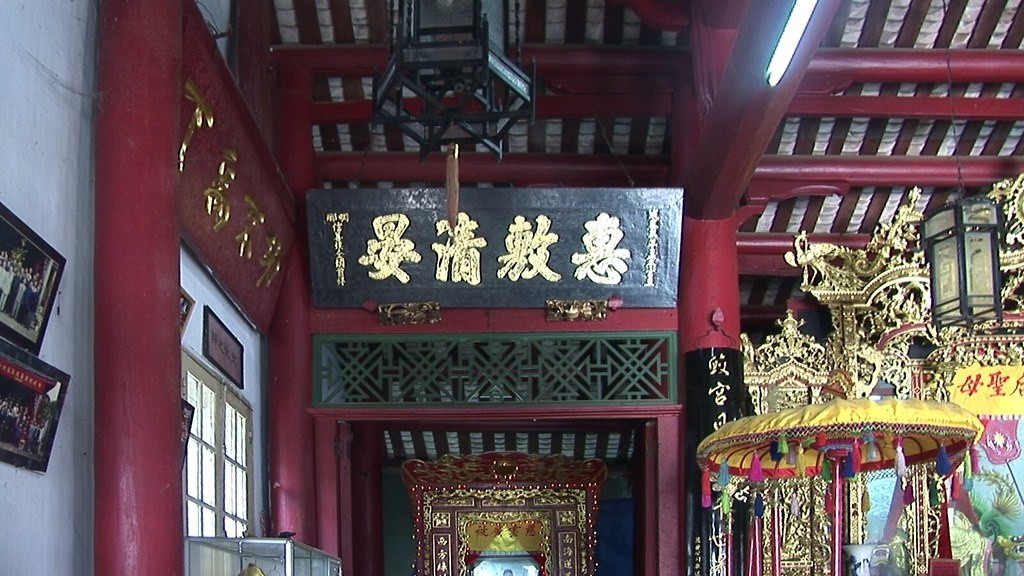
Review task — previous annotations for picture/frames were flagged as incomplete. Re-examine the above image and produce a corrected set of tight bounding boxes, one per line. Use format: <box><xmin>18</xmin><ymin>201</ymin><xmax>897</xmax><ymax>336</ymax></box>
<box><xmin>0</xmin><ymin>201</ymin><xmax>67</xmax><ymax>357</ymax></box>
<box><xmin>181</xmin><ymin>395</ymin><xmax>195</xmax><ymax>467</ymax></box>
<box><xmin>179</xmin><ymin>286</ymin><xmax>196</xmax><ymax>337</ymax></box>
<box><xmin>0</xmin><ymin>339</ymin><xmax>71</xmax><ymax>472</ymax></box>
<box><xmin>201</xmin><ymin>305</ymin><xmax>244</xmax><ymax>387</ymax></box>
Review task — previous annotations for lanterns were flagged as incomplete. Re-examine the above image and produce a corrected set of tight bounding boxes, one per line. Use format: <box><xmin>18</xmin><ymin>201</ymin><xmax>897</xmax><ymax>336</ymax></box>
<box><xmin>922</xmin><ymin>200</ymin><xmax>1004</xmax><ymax>334</ymax></box>
<box><xmin>372</xmin><ymin>0</ymin><xmax>536</xmax><ymax>232</ymax></box>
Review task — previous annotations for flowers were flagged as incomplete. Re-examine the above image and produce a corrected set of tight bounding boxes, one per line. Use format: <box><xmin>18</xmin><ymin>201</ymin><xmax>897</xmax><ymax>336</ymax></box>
<box><xmin>843</xmin><ymin>543</ymin><xmax>877</xmax><ymax>576</ymax></box>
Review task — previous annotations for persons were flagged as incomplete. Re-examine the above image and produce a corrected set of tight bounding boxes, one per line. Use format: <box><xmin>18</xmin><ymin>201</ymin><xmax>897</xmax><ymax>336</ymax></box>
<box><xmin>0</xmin><ymin>249</ymin><xmax>40</xmax><ymax>331</ymax></box>
<box><xmin>0</xmin><ymin>394</ymin><xmax>45</xmax><ymax>454</ymax></box>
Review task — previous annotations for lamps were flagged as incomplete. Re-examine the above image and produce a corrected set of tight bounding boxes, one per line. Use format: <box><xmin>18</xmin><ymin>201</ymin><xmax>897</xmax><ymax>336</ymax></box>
<box><xmin>764</xmin><ymin>0</ymin><xmax>818</xmax><ymax>86</ymax></box>
<box><xmin>918</xmin><ymin>0</ymin><xmax>1005</xmax><ymax>330</ymax></box>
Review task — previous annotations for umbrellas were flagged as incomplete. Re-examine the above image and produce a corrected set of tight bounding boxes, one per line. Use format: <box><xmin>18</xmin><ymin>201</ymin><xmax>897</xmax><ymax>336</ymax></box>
<box><xmin>697</xmin><ymin>395</ymin><xmax>985</xmax><ymax>575</ymax></box>
